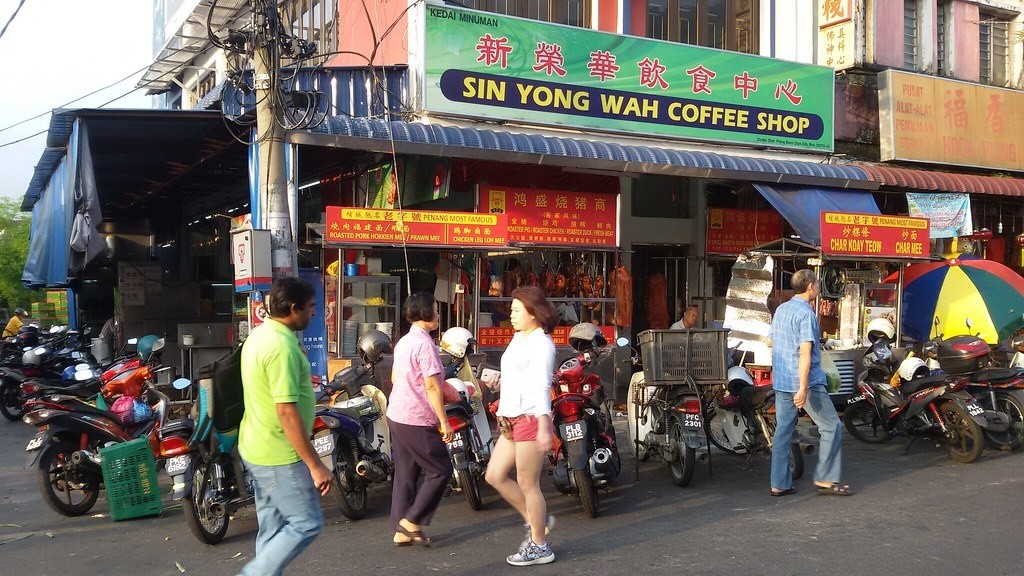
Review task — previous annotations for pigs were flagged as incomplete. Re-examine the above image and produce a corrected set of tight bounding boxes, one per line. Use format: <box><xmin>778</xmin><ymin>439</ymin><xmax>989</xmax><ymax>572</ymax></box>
<box><xmin>607</xmin><ymin>267</ymin><xmax>670</xmax><ymax>330</ymax></box>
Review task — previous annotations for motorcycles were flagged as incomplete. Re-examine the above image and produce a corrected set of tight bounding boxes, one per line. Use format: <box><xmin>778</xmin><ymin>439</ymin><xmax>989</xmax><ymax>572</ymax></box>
<box><xmin>435</xmin><ymin>338</ymin><xmax>491</xmax><ymax>508</ymax></box>
<box><xmin>616</xmin><ymin>337</ymin><xmax>706</xmax><ymax>488</ymax></box>
<box><xmin>923</xmin><ymin>313</ymin><xmax>1024</xmax><ymax>454</ymax></box>
<box><xmin>703</xmin><ymin>347</ymin><xmax>817</xmax><ymax>479</ymax></box>
<box><xmin>0</xmin><ymin>321</ymin><xmax>168</xmax><ymax>518</ymax></box>
<box><xmin>160</xmin><ymin>364</ymin><xmax>255</xmax><ymax>544</ymax></box>
<box><xmin>549</xmin><ymin>338</ymin><xmax>623</xmax><ymax>516</ymax></box>
<box><xmin>308</xmin><ymin>357</ymin><xmax>397</xmax><ymax>518</ymax></box>
<box><xmin>843</xmin><ymin>318</ymin><xmax>989</xmax><ymax>463</ymax></box>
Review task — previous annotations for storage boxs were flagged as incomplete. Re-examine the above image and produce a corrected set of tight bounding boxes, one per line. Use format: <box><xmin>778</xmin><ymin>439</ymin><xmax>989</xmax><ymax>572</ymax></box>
<box><xmin>636</xmin><ymin>328</ymin><xmax>732</xmax><ymax>386</ymax></box>
<box><xmin>935</xmin><ymin>333</ymin><xmax>990</xmax><ymax>374</ymax></box>
<box><xmin>97</xmin><ymin>433</ymin><xmax>163</xmax><ymax>523</ymax></box>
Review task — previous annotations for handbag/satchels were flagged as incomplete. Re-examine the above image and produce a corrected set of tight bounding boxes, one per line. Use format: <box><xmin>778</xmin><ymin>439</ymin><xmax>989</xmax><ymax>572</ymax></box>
<box><xmin>495</xmin><ymin>416</ymin><xmax>521</xmax><ymax>439</ymax></box>
<box><xmin>186</xmin><ymin>337</ymin><xmax>248</xmax><ymax>454</ymax></box>
<box><xmin>96</xmin><ymin>392</ymin><xmax>108</xmax><ymax>411</ymax></box>
<box><xmin>110</xmin><ymin>396</ymin><xmax>153</xmax><ymax>427</ymax></box>
<box><xmin>488</xmin><ymin>273</ymin><xmax>505</xmax><ymax>297</ymax></box>
<box><xmin>890</xmin><ymin>351</ymin><xmax>916</xmax><ymax>388</ymax></box>
<box><xmin>453</xmin><ymin>270</ymin><xmax>472</xmax><ymax>314</ymax></box>
<box><xmin>818</xmin><ymin>345</ymin><xmax>841</xmax><ymax>394</ymax></box>
<box><xmin>433</xmin><ymin>279</ymin><xmax>455</xmax><ymax>305</ymax></box>
<box><xmin>458</xmin><ymin>356</ymin><xmax>482</xmax><ymax>399</ymax></box>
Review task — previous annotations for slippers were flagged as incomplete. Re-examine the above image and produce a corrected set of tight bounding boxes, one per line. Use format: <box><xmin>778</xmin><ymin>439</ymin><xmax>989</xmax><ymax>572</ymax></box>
<box><xmin>770</xmin><ymin>487</ymin><xmax>798</xmax><ymax>496</ymax></box>
<box><xmin>816</xmin><ymin>482</ymin><xmax>855</xmax><ymax>496</ymax></box>
<box><xmin>393</xmin><ymin>524</ymin><xmax>431</xmax><ymax>547</ymax></box>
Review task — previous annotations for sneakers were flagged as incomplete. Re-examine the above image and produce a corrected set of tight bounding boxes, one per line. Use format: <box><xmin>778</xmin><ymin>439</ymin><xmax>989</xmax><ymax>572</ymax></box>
<box><xmin>505</xmin><ymin>540</ymin><xmax>555</xmax><ymax>566</ymax></box>
<box><xmin>518</xmin><ymin>515</ymin><xmax>554</xmax><ymax>552</ymax></box>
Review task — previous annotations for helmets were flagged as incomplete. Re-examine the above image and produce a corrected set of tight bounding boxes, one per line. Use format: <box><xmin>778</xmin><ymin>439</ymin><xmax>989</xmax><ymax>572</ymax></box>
<box><xmin>22</xmin><ymin>350</ymin><xmax>40</xmax><ymax>366</ymax></box>
<box><xmin>441</xmin><ymin>327</ymin><xmax>478</xmax><ymax>358</ymax></box>
<box><xmin>65</xmin><ymin>327</ymin><xmax>81</xmax><ymax>340</ymax></box>
<box><xmin>356</xmin><ymin>329</ymin><xmax>394</xmax><ymax>362</ymax></box>
<box><xmin>136</xmin><ymin>335</ymin><xmax>159</xmax><ymax>362</ymax></box>
<box><xmin>866</xmin><ymin>318</ymin><xmax>895</xmax><ymax>344</ymax></box>
<box><xmin>899</xmin><ymin>357</ymin><xmax>930</xmax><ymax>382</ymax></box>
<box><xmin>724</xmin><ymin>366</ymin><xmax>754</xmax><ymax>396</ymax></box>
<box><xmin>568</xmin><ymin>323</ymin><xmax>607</xmax><ymax>351</ymax></box>
<box><xmin>61</xmin><ymin>365</ymin><xmax>76</xmax><ymax>383</ymax></box>
<box><xmin>14</xmin><ymin>308</ymin><xmax>23</xmax><ymax>315</ymax></box>
<box><xmin>444</xmin><ymin>378</ymin><xmax>469</xmax><ymax>403</ymax></box>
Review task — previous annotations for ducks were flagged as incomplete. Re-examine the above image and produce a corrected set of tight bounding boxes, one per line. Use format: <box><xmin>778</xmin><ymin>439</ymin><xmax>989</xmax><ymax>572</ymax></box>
<box><xmin>488</xmin><ymin>251</ymin><xmax>604</xmax><ymax>311</ymax></box>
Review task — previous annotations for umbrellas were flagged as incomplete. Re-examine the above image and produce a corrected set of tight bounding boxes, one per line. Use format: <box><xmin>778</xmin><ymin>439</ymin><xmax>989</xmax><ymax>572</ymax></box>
<box><xmin>868</xmin><ymin>253</ymin><xmax>1024</xmax><ymax>349</ymax></box>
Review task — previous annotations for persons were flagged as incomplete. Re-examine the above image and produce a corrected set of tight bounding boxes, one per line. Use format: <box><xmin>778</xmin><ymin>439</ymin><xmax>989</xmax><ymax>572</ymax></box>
<box><xmin>490</xmin><ymin>258</ymin><xmax>522</xmax><ymax>327</ymax></box>
<box><xmin>766</xmin><ymin>269</ymin><xmax>854</xmax><ymax>495</ymax></box>
<box><xmin>669</xmin><ymin>306</ymin><xmax>698</xmax><ymax>330</ymax></box>
<box><xmin>386</xmin><ymin>291</ymin><xmax>454</xmax><ymax>546</ymax></box>
<box><xmin>233</xmin><ymin>276</ymin><xmax>335</xmax><ymax>576</ymax></box>
<box><xmin>99</xmin><ymin>317</ymin><xmax>114</xmax><ymax>339</ymax></box>
<box><xmin>485</xmin><ymin>286</ymin><xmax>556</xmax><ymax>566</ymax></box>
<box><xmin>2</xmin><ymin>308</ymin><xmax>29</xmax><ymax>340</ymax></box>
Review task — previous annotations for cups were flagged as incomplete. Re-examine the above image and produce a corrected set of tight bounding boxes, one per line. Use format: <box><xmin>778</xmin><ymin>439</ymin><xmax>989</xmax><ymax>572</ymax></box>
<box><xmin>872</xmin><ymin>300</ymin><xmax>877</xmax><ymax>307</ymax></box>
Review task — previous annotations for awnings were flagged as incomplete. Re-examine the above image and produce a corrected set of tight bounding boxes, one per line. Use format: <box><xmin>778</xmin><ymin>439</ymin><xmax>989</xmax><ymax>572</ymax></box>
<box><xmin>19</xmin><ymin>107</ymin><xmax>249</xmax><ymax>212</ymax></box>
<box><xmin>292</xmin><ymin>114</ymin><xmax>1024</xmax><ymax>200</ymax></box>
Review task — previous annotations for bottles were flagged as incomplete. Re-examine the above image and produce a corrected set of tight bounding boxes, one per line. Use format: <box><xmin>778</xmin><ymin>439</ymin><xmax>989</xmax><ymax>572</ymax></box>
<box><xmin>835</xmin><ymin>329</ymin><xmax>840</xmax><ymax>340</ymax></box>
<box><xmin>823</xmin><ymin>331</ymin><xmax>827</xmax><ymax>338</ymax></box>
